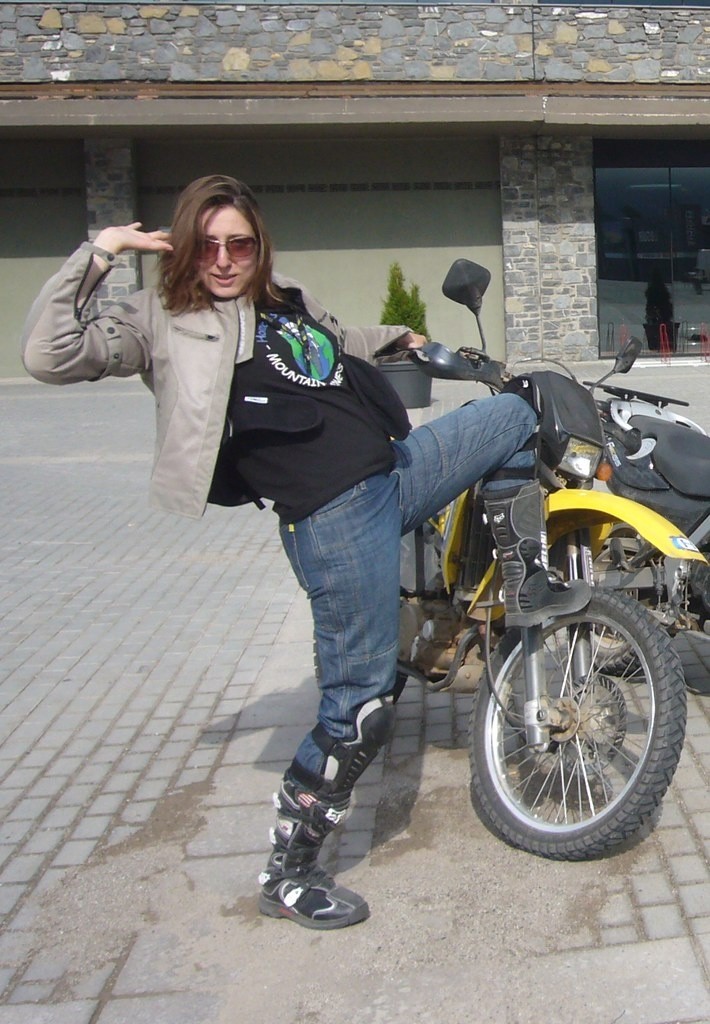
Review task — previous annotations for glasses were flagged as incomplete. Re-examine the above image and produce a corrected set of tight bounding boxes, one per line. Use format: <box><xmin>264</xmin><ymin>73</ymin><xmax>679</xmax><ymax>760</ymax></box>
<box><xmin>192</xmin><ymin>236</ymin><xmax>259</xmax><ymax>261</ymax></box>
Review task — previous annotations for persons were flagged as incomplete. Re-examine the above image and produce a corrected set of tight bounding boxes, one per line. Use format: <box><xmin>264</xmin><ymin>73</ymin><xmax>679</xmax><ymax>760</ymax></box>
<box><xmin>22</xmin><ymin>174</ymin><xmax>594</xmax><ymax>926</ymax></box>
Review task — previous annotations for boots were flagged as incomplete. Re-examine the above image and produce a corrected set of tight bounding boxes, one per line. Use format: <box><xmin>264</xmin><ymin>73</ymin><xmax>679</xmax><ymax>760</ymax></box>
<box><xmin>260</xmin><ymin>778</ymin><xmax>370</xmax><ymax>929</ymax></box>
<box><xmin>482</xmin><ymin>478</ymin><xmax>592</xmax><ymax>628</ymax></box>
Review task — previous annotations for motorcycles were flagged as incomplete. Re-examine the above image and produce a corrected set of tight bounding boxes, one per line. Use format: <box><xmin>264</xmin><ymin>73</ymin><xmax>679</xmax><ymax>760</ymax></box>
<box><xmin>391</xmin><ymin>257</ymin><xmax>710</xmax><ymax>862</ymax></box>
<box><xmin>580</xmin><ymin>376</ymin><xmax>710</xmax><ymax>676</ymax></box>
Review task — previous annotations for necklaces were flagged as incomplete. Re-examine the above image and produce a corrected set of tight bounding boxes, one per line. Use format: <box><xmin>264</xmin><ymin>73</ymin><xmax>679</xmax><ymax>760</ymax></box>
<box><xmin>255</xmin><ymin>302</ymin><xmax>314</xmax><ymax>375</ymax></box>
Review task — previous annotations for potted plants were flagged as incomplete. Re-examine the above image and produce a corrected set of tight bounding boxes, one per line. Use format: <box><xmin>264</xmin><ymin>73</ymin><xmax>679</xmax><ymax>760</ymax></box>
<box><xmin>375</xmin><ymin>261</ymin><xmax>433</xmax><ymax>409</ymax></box>
